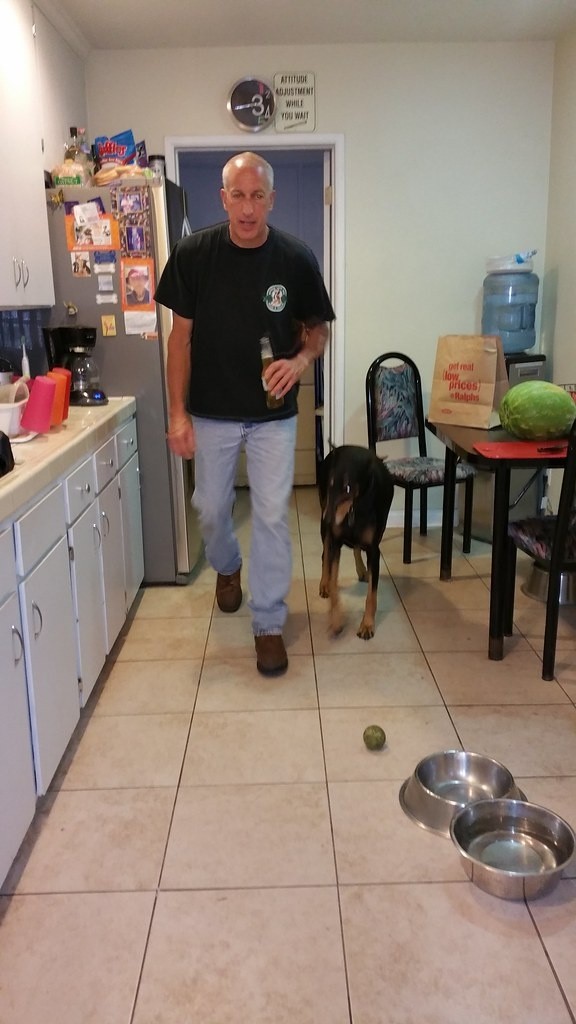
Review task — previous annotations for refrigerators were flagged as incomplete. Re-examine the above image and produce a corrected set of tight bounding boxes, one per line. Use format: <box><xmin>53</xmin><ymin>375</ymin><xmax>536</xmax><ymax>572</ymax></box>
<box><xmin>43</xmin><ymin>178</ymin><xmax>205</xmax><ymax>585</ymax></box>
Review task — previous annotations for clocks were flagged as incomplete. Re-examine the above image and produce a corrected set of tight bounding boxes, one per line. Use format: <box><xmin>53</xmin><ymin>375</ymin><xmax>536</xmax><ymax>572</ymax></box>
<box><xmin>226</xmin><ymin>75</ymin><xmax>278</xmax><ymax>133</ymax></box>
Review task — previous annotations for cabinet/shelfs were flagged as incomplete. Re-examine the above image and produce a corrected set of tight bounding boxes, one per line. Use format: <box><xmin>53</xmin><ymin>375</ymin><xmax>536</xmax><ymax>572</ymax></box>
<box><xmin>1</xmin><ymin>396</ymin><xmax>145</xmax><ymax>893</ymax></box>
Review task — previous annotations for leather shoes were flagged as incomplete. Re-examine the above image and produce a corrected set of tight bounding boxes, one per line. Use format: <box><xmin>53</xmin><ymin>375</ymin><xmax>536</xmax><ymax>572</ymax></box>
<box><xmin>253</xmin><ymin>634</ymin><xmax>289</xmax><ymax>677</ymax></box>
<box><xmin>216</xmin><ymin>558</ymin><xmax>243</xmax><ymax>613</ymax></box>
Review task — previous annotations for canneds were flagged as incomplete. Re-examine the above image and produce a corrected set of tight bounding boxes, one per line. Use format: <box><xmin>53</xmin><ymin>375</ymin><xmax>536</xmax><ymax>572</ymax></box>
<box><xmin>148</xmin><ymin>155</ymin><xmax>166</xmax><ymax>177</ymax></box>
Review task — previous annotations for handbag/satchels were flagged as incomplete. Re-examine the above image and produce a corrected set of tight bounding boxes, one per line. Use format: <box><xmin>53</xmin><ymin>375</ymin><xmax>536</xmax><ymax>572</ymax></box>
<box><xmin>427</xmin><ymin>332</ymin><xmax>509</xmax><ymax>430</ymax></box>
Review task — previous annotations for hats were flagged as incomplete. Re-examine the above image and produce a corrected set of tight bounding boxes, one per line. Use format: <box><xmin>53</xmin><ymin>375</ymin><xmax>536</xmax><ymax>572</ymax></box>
<box><xmin>126</xmin><ymin>269</ymin><xmax>149</xmax><ymax>285</ymax></box>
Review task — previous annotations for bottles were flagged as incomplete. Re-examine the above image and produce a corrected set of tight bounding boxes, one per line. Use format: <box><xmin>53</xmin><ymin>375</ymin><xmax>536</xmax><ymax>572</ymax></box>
<box><xmin>73</xmin><ymin>355</ymin><xmax>88</xmax><ymax>391</ymax></box>
<box><xmin>64</xmin><ymin>126</ymin><xmax>81</xmax><ymax>165</ymax></box>
<box><xmin>85</xmin><ymin>354</ymin><xmax>100</xmax><ymax>390</ymax></box>
<box><xmin>91</xmin><ymin>144</ymin><xmax>100</xmax><ymax>173</ymax></box>
<box><xmin>147</xmin><ymin>153</ymin><xmax>165</xmax><ymax>176</ymax></box>
<box><xmin>75</xmin><ymin>128</ymin><xmax>93</xmax><ymax>177</ymax></box>
<box><xmin>500</xmin><ymin>248</ymin><xmax>538</xmax><ymax>266</ymax></box>
<box><xmin>481</xmin><ymin>274</ymin><xmax>539</xmax><ymax>356</ymax></box>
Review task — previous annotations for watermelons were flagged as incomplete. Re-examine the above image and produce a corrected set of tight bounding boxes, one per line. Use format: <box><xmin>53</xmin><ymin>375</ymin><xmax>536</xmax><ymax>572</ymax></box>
<box><xmin>499</xmin><ymin>380</ymin><xmax>576</xmax><ymax>440</ymax></box>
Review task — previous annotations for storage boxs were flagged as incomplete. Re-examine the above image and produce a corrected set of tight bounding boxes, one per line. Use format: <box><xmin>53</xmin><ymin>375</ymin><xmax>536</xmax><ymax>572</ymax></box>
<box><xmin>397</xmin><ymin>747</ymin><xmax>533</xmax><ymax>842</ymax></box>
<box><xmin>450</xmin><ymin>797</ymin><xmax>576</xmax><ymax>902</ymax></box>
<box><xmin>43</xmin><ymin>322</ymin><xmax>109</xmax><ymax>407</ymax></box>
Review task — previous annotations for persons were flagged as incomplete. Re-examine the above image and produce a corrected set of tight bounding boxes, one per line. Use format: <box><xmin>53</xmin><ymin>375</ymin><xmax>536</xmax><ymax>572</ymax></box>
<box><xmin>72</xmin><ymin>193</ymin><xmax>152</xmax><ymax>307</ymax></box>
<box><xmin>151</xmin><ymin>151</ymin><xmax>337</xmax><ymax>678</ymax></box>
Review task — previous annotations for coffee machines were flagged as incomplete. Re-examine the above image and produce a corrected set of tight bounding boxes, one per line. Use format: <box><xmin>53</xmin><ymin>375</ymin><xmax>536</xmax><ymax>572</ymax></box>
<box><xmin>42</xmin><ymin>323</ymin><xmax>108</xmax><ymax>405</ymax></box>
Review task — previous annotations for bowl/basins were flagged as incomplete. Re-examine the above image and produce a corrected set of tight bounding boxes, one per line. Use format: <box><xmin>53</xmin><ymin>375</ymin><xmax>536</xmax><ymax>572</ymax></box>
<box><xmin>402</xmin><ymin>749</ymin><xmax>529</xmax><ymax>839</ymax></box>
<box><xmin>451</xmin><ymin>797</ymin><xmax>574</xmax><ymax>901</ymax></box>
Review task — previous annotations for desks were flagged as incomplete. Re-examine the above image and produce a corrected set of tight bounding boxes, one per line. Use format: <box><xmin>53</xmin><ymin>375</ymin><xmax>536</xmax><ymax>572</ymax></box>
<box><xmin>422</xmin><ymin>411</ymin><xmax>576</xmax><ymax>663</ymax></box>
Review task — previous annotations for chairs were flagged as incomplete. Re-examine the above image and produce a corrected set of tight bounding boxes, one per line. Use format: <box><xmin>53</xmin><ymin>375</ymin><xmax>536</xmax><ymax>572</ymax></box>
<box><xmin>504</xmin><ymin>418</ymin><xmax>576</xmax><ymax>682</ymax></box>
<box><xmin>365</xmin><ymin>350</ymin><xmax>477</xmax><ymax>564</ymax></box>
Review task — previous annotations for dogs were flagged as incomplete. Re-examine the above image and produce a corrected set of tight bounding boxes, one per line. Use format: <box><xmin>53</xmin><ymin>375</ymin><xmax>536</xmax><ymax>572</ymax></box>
<box><xmin>316</xmin><ymin>439</ymin><xmax>396</xmax><ymax>641</ymax></box>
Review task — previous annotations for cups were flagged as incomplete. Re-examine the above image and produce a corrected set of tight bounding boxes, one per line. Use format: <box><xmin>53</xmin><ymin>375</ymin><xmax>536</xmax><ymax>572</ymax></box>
<box><xmin>51</xmin><ymin>368</ymin><xmax>71</xmax><ymax>419</ymax></box>
<box><xmin>46</xmin><ymin>371</ymin><xmax>67</xmax><ymax>424</ymax></box>
<box><xmin>19</xmin><ymin>376</ymin><xmax>55</xmax><ymax>433</ymax></box>
